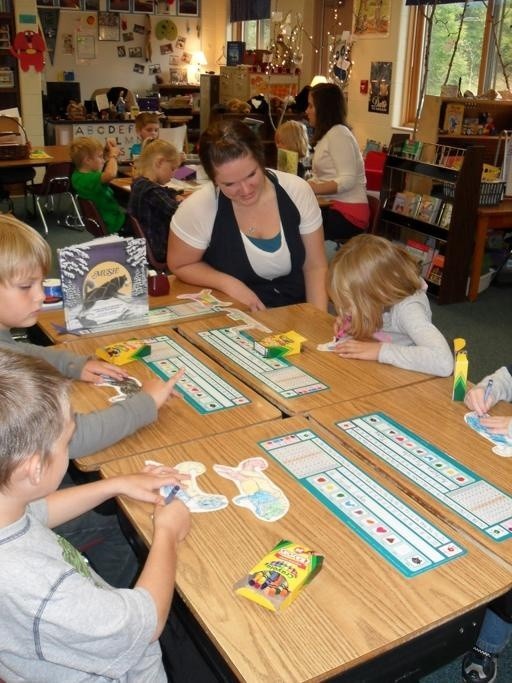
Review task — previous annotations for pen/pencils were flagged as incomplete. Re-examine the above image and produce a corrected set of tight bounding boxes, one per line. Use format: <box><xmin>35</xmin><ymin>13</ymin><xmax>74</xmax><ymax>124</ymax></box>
<box><xmin>149</xmin><ymin>486</ymin><xmax>180</xmax><ymax>517</ymax></box>
<box><xmin>477</xmin><ymin>380</ymin><xmax>493</xmax><ymax>418</ymax></box>
<box><xmin>336</xmin><ymin>316</ymin><xmax>352</xmax><ymax>342</ymax></box>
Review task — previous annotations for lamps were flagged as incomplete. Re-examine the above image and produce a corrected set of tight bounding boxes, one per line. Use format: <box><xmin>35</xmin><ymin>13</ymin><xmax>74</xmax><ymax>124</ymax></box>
<box><xmin>188</xmin><ymin>49</ymin><xmax>208</xmax><ymax>73</ymax></box>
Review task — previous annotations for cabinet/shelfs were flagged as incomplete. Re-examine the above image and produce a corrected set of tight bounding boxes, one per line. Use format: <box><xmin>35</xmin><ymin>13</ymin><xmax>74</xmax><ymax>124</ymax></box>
<box><xmin>198</xmin><ymin>70</ymin><xmax>219</xmax><ymax>135</ymax></box>
<box><xmin>399</xmin><ymin>89</ymin><xmax>512</xmax><ymax>247</ymax></box>
<box><xmin>219</xmin><ymin>64</ymin><xmax>251</xmax><ymax>105</ymax></box>
<box><xmin>368</xmin><ymin>134</ymin><xmax>483</xmax><ymax>305</ymax></box>
<box><xmin>0</xmin><ymin>0</ymin><xmax>24</xmax><ymax>117</ymax></box>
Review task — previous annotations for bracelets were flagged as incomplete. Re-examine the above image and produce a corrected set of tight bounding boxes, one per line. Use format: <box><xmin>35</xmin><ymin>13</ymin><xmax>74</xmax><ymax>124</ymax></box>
<box><xmin>111</xmin><ymin>153</ymin><xmax>119</xmax><ymax>162</ymax></box>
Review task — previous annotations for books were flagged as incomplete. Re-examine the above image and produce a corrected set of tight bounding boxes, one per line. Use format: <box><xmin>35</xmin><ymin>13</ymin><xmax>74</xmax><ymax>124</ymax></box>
<box><xmin>277</xmin><ymin>149</ymin><xmax>297</xmax><ymax>177</ymax></box>
<box><xmin>389</xmin><ymin>191</ymin><xmax>452</xmax><ymax>285</ymax></box>
<box><xmin>57</xmin><ymin>234</ymin><xmax>149</xmax><ymax>333</ymax></box>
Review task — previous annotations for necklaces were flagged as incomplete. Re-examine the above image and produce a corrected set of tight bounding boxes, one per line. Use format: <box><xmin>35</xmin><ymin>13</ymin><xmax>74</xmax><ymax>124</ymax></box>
<box><xmin>237</xmin><ymin>210</ymin><xmax>267</xmax><ymax>235</ymax></box>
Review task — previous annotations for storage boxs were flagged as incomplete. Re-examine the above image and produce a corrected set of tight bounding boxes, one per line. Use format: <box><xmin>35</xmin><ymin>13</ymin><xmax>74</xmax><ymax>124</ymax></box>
<box><xmin>444</xmin><ymin>181</ymin><xmax>506</xmax><ymax>207</ymax></box>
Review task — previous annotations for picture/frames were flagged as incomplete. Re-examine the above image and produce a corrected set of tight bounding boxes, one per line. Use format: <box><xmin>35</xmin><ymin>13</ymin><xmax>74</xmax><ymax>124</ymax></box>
<box><xmin>36</xmin><ymin>0</ymin><xmax>200</xmax><ymax>17</ymax></box>
<box><xmin>96</xmin><ymin>9</ymin><xmax>122</xmax><ymax>41</ymax></box>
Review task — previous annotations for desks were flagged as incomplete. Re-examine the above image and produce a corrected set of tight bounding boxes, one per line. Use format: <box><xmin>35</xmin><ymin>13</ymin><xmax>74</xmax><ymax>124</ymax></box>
<box><xmin>109</xmin><ymin>165</ymin><xmax>200</xmax><ymax>211</ymax></box>
<box><xmin>248</xmin><ymin>69</ymin><xmax>301</xmax><ymax>98</ymax></box>
<box><xmin>35</xmin><ymin>273</ymin><xmax>511</xmax><ymax>683</ymax></box>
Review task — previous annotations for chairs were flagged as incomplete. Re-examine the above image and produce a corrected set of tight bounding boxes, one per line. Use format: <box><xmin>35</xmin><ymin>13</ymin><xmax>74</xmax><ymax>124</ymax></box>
<box><xmin>77</xmin><ymin>197</ymin><xmax>107</xmax><ymax>238</ymax></box>
<box><xmin>123</xmin><ymin>213</ymin><xmax>167</xmax><ymax>271</ymax></box>
<box><xmin>1</xmin><ymin>144</ymin><xmax>84</xmax><ymax>238</ymax></box>
<box><xmin>324</xmin><ymin>194</ymin><xmax>381</xmax><ymax>247</ymax></box>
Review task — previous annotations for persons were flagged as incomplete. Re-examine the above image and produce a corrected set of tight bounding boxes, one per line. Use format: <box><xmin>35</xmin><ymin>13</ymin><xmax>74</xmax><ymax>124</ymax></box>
<box><xmin>276</xmin><ymin>120</ymin><xmax>308</xmax><ymax>158</ymax></box>
<box><xmin>466</xmin><ymin>361</ymin><xmax>512</xmax><ymax>439</ymax></box>
<box><xmin>304</xmin><ymin>83</ymin><xmax>369</xmax><ymax>244</ymax></box>
<box><xmin>128</xmin><ymin>137</ymin><xmax>184</xmax><ymax>263</ymax></box>
<box><xmin>0</xmin><ymin>213</ymin><xmax>184</xmax><ymax>582</ymax></box>
<box><xmin>168</xmin><ymin>121</ymin><xmax>328</xmax><ymax>312</ymax></box>
<box><xmin>69</xmin><ymin>137</ymin><xmax>126</xmax><ymax>235</ymax></box>
<box><xmin>130</xmin><ymin>113</ymin><xmax>160</xmax><ymax>163</ymax></box>
<box><xmin>0</xmin><ymin>347</ymin><xmax>191</xmax><ymax>683</ymax></box>
<box><xmin>325</xmin><ymin>234</ymin><xmax>452</xmax><ymax>375</ymax></box>
